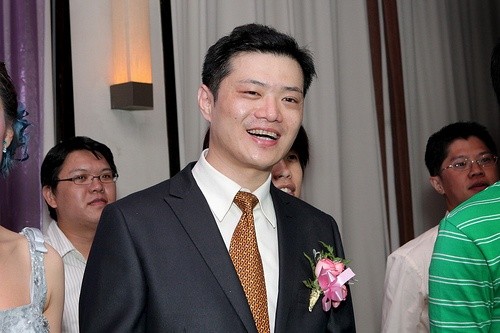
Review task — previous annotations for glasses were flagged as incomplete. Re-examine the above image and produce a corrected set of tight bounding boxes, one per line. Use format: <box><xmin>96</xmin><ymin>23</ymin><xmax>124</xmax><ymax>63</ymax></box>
<box><xmin>438</xmin><ymin>154</ymin><xmax>499</xmax><ymax>173</ymax></box>
<box><xmin>50</xmin><ymin>172</ymin><xmax>119</xmax><ymax>185</ymax></box>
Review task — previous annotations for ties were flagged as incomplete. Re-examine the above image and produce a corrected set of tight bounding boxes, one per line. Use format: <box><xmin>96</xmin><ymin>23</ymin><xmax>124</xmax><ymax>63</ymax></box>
<box><xmin>229</xmin><ymin>191</ymin><xmax>270</xmax><ymax>333</ymax></box>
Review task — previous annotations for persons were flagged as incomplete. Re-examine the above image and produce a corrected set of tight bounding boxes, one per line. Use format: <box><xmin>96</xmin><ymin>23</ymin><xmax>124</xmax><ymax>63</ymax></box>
<box><xmin>0</xmin><ymin>61</ymin><xmax>65</xmax><ymax>333</ymax></box>
<box><xmin>39</xmin><ymin>135</ymin><xmax>120</xmax><ymax>333</ymax></box>
<box><xmin>425</xmin><ymin>180</ymin><xmax>500</xmax><ymax>333</ymax></box>
<box><xmin>380</xmin><ymin>120</ymin><xmax>500</xmax><ymax>333</ymax></box>
<box><xmin>202</xmin><ymin>121</ymin><xmax>310</xmax><ymax>198</ymax></box>
<box><xmin>76</xmin><ymin>22</ymin><xmax>357</xmax><ymax>333</ymax></box>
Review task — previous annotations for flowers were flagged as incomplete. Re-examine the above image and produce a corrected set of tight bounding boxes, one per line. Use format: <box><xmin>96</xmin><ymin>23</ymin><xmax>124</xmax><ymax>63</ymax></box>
<box><xmin>301</xmin><ymin>240</ymin><xmax>360</xmax><ymax>313</ymax></box>
